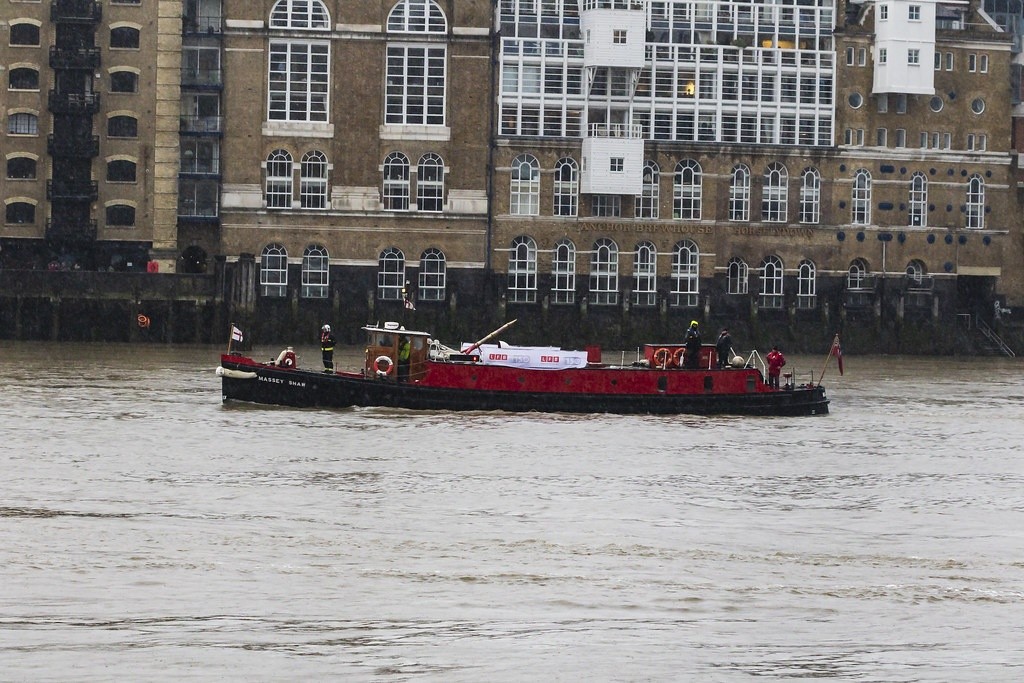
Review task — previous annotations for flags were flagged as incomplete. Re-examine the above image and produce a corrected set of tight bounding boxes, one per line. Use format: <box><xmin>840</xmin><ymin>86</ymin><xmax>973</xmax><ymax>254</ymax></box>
<box><xmin>232</xmin><ymin>326</ymin><xmax>243</xmax><ymax>342</ymax></box>
<box><xmin>403</xmin><ymin>297</ymin><xmax>416</xmax><ymax>310</ymax></box>
<box><xmin>833</xmin><ymin>337</ymin><xmax>843</xmax><ymax>376</ymax></box>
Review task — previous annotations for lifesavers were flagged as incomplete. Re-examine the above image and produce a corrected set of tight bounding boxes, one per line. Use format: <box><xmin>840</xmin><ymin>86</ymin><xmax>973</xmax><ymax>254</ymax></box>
<box><xmin>673</xmin><ymin>347</ymin><xmax>688</xmax><ymax>368</ymax></box>
<box><xmin>653</xmin><ymin>348</ymin><xmax>673</xmax><ymax>368</ymax></box>
<box><xmin>373</xmin><ymin>355</ymin><xmax>394</xmax><ymax>377</ymax></box>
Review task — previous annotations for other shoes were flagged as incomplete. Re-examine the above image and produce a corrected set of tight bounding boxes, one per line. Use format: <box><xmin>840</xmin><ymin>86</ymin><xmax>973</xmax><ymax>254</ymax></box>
<box><xmin>322</xmin><ymin>370</ymin><xmax>333</xmax><ymax>374</ymax></box>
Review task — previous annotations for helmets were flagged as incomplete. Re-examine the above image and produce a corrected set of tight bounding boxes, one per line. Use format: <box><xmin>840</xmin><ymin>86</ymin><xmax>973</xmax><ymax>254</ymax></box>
<box><xmin>322</xmin><ymin>325</ymin><xmax>331</xmax><ymax>332</ymax></box>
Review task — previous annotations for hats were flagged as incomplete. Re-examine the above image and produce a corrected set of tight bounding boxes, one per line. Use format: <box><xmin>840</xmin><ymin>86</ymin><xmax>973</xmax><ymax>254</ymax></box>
<box><xmin>773</xmin><ymin>346</ymin><xmax>778</xmax><ymax>350</ymax></box>
<box><xmin>690</xmin><ymin>321</ymin><xmax>698</xmax><ymax>328</ymax></box>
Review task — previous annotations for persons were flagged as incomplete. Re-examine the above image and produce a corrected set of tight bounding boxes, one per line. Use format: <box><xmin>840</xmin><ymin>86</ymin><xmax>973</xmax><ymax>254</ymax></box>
<box><xmin>398</xmin><ymin>335</ymin><xmax>410</xmax><ymax>382</ymax></box>
<box><xmin>684</xmin><ymin>320</ymin><xmax>701</xmax><ymax>369</ymax></box>
<box><xmin>766</xmin><ymin>346</ymin><xmax>786</xmax><ymax>389</ymax></box>
<box><xmin>715</xmin><ymin>328</ymin><xmax>733</xmax><ymax>369</ymax></box>
<box><xmin>322</xmin><ymin>325</ymin><xmax>336</xmax><ymax>374</ymax></box>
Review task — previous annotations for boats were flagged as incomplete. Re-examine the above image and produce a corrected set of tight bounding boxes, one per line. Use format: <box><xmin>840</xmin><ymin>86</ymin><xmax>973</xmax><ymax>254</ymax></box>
<box><xmin>216</xmin><ymin>319</ymin><xmax>830</xmax><ymax>416</ymax></box>
